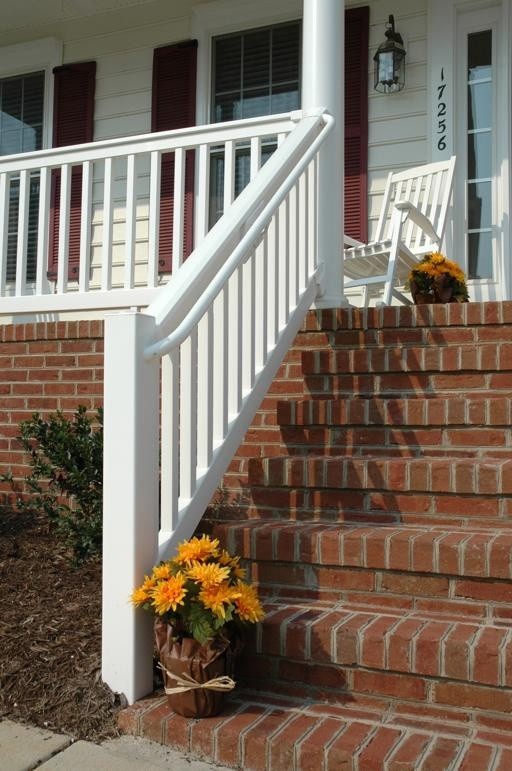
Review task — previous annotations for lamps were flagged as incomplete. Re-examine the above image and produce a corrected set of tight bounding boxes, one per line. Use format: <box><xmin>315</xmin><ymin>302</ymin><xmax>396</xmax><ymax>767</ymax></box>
<box><xmin>373</xmin><ymin>15</ymin><xmax>407</xmax><ymax>94</ymax></box>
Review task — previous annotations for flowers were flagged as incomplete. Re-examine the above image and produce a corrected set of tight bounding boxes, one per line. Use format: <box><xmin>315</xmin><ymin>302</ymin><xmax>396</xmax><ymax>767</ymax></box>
<box><xmin>408</xmin><ymin>250</ymin><xmax>470</xmax><ymax>303</ymax></box>
<box><xmin>128</xmin><ymin>533</ymin><xmax>266</xmax><ymax>646</ymax></box>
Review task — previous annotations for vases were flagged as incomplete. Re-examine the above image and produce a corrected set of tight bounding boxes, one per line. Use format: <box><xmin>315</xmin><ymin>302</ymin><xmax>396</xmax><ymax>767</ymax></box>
<box><xmin>410</xmin><ymin>274</ymin><xmax>454</xmax><ymax>304</ymax></box>
<box><xmin>154</xmin><ymin>617</ymin><xmax>248</xmax><ymax>718</ymax></box>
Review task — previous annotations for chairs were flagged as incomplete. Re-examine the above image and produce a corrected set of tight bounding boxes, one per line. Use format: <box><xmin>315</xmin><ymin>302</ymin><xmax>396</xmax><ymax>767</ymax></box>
<box><xmin>344</xmin><ymin>155</ymin><xmax>456</xmax><ymax>308</ymax></box>
